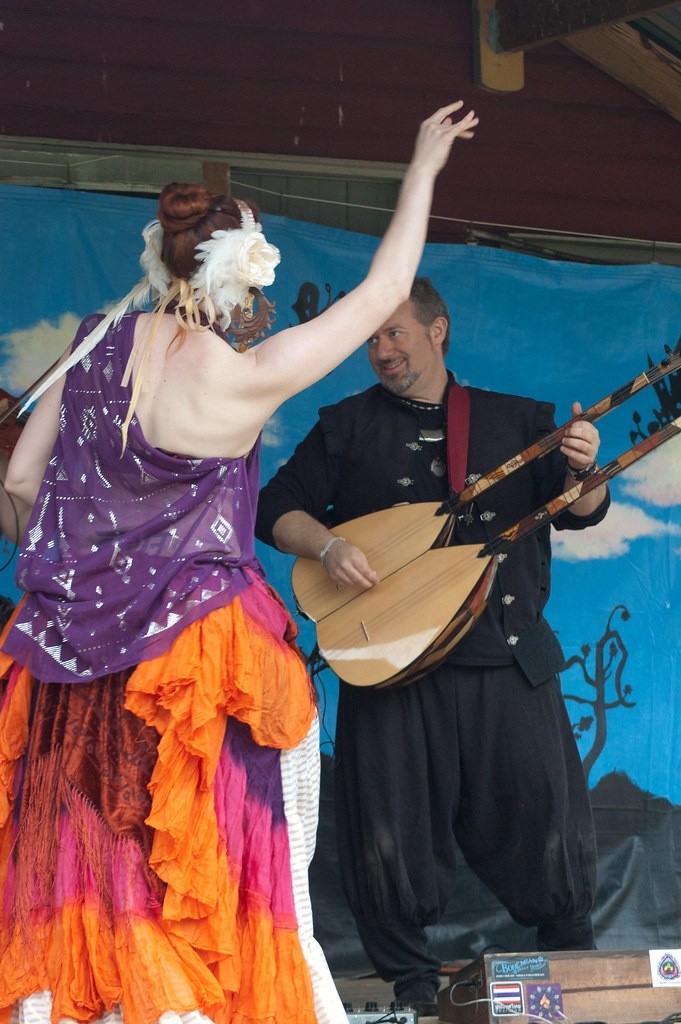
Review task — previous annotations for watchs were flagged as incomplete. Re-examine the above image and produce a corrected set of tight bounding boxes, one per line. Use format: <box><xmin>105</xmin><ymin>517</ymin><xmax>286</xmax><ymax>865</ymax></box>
<box><xmin>564</xmin><ymin>456</ymin><xmax>597</xmax><ymax>481</ymax></box>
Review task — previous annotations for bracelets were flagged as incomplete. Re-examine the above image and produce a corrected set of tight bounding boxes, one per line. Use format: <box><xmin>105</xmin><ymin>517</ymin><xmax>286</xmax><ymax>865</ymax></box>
<box><xmin>320</xmin><ymin>536</ymin><xmax>346</xmax><ymax>567</ymax></box>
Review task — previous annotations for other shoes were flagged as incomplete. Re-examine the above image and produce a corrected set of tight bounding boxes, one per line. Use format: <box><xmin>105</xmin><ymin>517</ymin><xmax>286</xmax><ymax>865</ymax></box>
<box><xmin>395</xmin><ymin>981</ymin><xmax>440</xmax><ymax>1016</ymax></box>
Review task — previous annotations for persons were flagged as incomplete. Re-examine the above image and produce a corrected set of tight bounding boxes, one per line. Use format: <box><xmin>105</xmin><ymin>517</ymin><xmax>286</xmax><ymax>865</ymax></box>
<box><xmin>0</xmin><ymin>101</ymin><xmax>480</xmax><ymax>1023</ymax></box>
<box><xmin>253</xmin><ymin>278</ymin><xmax>611</xmax><ymax>1016</ymax></box>
